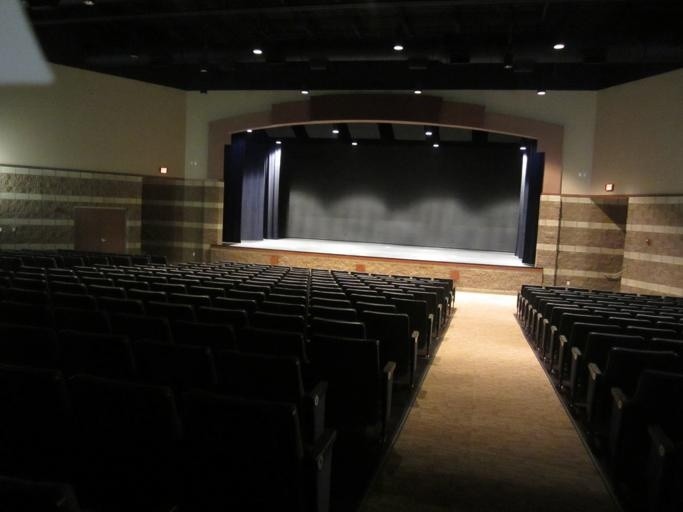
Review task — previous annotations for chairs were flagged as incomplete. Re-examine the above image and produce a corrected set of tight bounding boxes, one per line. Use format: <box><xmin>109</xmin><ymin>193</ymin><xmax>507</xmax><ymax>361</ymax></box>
<box><xmin>0</xmin><ymin>249</ymin><xmax>458</xmax><ymax>512</ymax></box>
<box><xmin>518</xmin><ymin>284</ymin><xmax>683</xmax><ymax>512</ymax></box>
